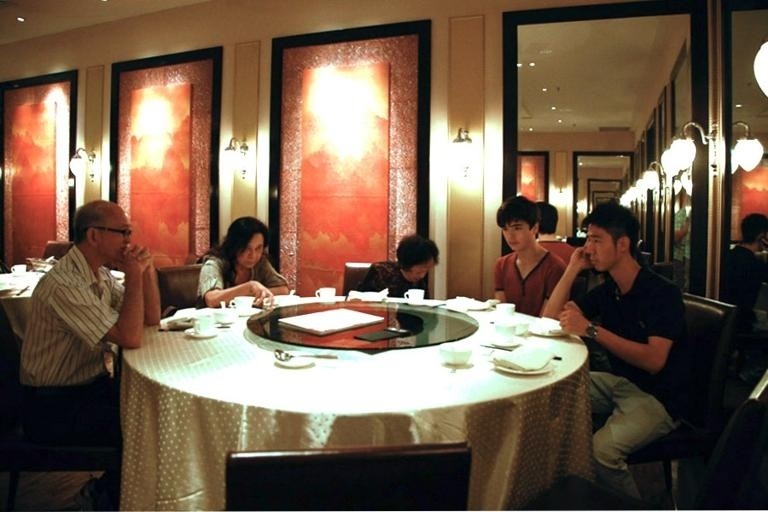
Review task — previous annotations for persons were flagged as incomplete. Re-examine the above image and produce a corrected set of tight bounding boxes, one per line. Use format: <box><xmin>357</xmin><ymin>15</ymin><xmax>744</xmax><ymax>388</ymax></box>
<box><xmin>539</xmin><ymin>202</ymin><xmax>691</xmax><ymax>509</ymax></box>
<box><xmin>353</xmin><ymin>232</ymin><xmax>441</xmax><ymax>298</ymax></box>
<box><xmin>673</xmin><ymin>187</ymin><xmax>692</xmax><ymax>272</ymax></box>
<box><xmin>16</xmin><ymin>200</ymin><xmax>164</xmax><ymax>511</ymax></box>
<box><xmin>194</xmin><ymin>216</ymin><xmax>293</xmax><ymax>313</ymax></box>
<box><xmin>490</xmin><ymin>196</ymin><xmax>569</xmax><ymax>320</ymax></box>
<box><xmin>724</xmin><ymin>212</ymin><xmax>768</xmax><ymax>383</ymax></box>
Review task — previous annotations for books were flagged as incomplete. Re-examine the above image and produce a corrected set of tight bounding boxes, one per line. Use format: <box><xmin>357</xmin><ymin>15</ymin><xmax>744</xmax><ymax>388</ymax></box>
<box><xmin>277</xmin><ymin>307</ymin><xmax>385</xmax><ymax>336</ymax></box>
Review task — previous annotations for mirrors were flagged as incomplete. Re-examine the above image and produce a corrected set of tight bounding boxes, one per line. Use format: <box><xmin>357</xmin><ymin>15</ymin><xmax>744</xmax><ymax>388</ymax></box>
<box><xmin>570</xmin><ymin>148</ymin><xmax>634</xmax><ymax>244</ymax></box>
<box><xmin>718</xmin><ymin>0</ymin><xmax>766</xmax><ymax>374</ymax></box>
<box><xmin>499</xmin><ymin>1</ymin><xmax>714</xmax><ymax>306</ymax></box>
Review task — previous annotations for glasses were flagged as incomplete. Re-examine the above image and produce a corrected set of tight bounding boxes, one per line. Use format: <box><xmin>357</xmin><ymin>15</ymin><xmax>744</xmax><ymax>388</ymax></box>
<box><xmin>94</xmin><ymin>225</ymin><xmax>133</xmax><ymax>237</ymax></box>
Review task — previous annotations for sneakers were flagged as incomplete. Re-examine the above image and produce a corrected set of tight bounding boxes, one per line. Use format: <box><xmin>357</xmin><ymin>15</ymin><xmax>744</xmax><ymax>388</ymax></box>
<box><xmin>74</xmin><ymin>477</ymin><xmax>112</xmax><ymax>511</ymax></box>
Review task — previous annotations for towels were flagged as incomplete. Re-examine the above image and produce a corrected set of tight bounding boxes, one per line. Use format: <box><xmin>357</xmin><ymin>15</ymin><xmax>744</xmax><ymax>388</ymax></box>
<box><xmin>162</xmin><ymin>307</ymin><xmax>213</xmax><ymax>329</ymax></box>
<box><xmin>525</xmin><ymin>315</ymin><xmax>565</xmax><ymax>337</ymax></box>
<box><xmin>345</xmin><ymin>287</ymin><xmax>390</xmax><ymax>301</ymax></box>
<box><xmin>491</xmin><ymin>345</ymin><xmax>556</xmax><ymax>373</ymax></box>
<box><xmin>453</xmin><ymin>295</ymin><xmax>501</xmax><ymax>310</ymax></box>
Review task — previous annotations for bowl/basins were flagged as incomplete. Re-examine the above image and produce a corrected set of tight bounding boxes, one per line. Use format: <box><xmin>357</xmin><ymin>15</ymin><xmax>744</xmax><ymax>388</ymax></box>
<box><xmin>214</xmin><ymin>307</ymin><xmax>239</xmax><ymax>324</ymax></box>
<box><xmin>514</xmin><ymin>319</ymin><xmax>532</xmax><ymax>338</ymax></box>
<box><xmin>437</xmin><ymin>342</ymin><xmax>474</xmax><ymax>367</ymax></box>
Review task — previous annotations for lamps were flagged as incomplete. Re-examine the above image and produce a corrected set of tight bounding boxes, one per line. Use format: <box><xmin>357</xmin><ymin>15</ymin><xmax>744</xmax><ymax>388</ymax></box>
<box><xmin>617</xmin><ymin>120</ymin><xmax>720</xmax><ymax>211</ymax></box>
<box><xmin>449</xmin><ymin>124</ymin><xmax>476</xmax><ymax>181</ymax></box>
<box><xmin>221</xmin><ymin>134</ymin><xmax>251</xmax><ymax>180</ymax></box>
<box><xmin>731</xmin><ymin>102</ymin><xmax>765</xmax><ymax>176</ymax></box>
<box><xmin>69</xmin><ymin>144</ymin><xmax>99</xmax><ymax>186</ymax></box>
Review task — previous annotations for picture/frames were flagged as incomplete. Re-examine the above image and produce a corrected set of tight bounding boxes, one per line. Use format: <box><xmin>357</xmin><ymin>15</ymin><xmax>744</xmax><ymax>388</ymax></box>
<box><xmin>517</xmin><ymin>150</ymin><xmax>549</xmax><ymax>205</ymax></box>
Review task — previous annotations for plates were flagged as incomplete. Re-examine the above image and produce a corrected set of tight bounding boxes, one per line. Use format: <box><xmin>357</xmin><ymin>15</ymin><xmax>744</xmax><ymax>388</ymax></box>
<box><xmin>183</xmin><ymin>327</ymin><xmax>219</xmax><ymax>339</ymax></box>
<box><xmin>274</xmin><ymin>350</ymin><xmax>315</xmax><ymax>369</ymax></box>
<box><xmin>527</xmin><ymin>320</ymin><xmax>566</xmax><ymax>338</ymax></box>
<box><xmin>493</xmin><ymin>364</ymin><xmax>556</xmax><ymax>377</ymax></box>
<box><xmin>263</xmin><ymin>293</ymin><xmax>300</xmax><ymax>306</ymax></box>
<box><xmin>236</xmin><ymin>307</ymin><xmax>263</xmax><ymax>319</ymax></box>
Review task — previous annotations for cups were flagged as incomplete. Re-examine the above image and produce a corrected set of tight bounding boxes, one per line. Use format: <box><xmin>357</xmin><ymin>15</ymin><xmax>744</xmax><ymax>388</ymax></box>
<box><xmin>493</xmin><ymin>324</ymin><xmax>516</xmax><ymax>344</ymax></box>
<box><xmin>192</xmin><ymin>314</ymin><xmax>213</xmax><ymax>333</ymax></box>
<box><xmin>402</xmin><ymin>288</ymin><xmax>426</xmax><ymax>306</ymax></box>
<box><xmin>227</xmin><ymin>295</ymin><xmax>255</xmax><ymax>311</ymax></box>
<box><xmin>313</xmin><ymin>286</ymin><xmax>336</xmax><ymax>302</ymax></box>
<box><xmin>494</xmin><ymin>303</ymin><xmax>515</xmax><ymax>319</ymax></box>
<box><xmin>11</xmin><ymin>264</ymin><xmax>27</xmax><ymax>276</ymax></box>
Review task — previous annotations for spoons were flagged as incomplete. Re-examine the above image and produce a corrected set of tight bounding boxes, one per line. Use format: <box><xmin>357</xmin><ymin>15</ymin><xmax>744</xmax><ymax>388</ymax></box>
<box><xmin>273</xmin><ymin>348</ymin><xmax>338</xmax><ymax>362</ymax></box>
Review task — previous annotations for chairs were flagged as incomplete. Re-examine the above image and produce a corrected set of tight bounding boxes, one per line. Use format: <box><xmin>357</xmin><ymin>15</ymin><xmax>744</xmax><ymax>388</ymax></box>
<box><xmin>616</xmin><ymin>286</ymin><xmax>737</xmax><ymax>510</ymax></box>
<box><xmin>40</xmin><ymin>239</ymin><xmax>73</xmax><ymax>262</ymax></box>
<box><xmin>155</xmin><ymin>263</ymin><xmax>211</xmax><ymax>314</ymax></box>
<box><xmin>342</xmin><ymin>261</ymin><xmax>373</xmax><ymax>296</ymax></box>
<box><xmin>0</xmin><ymin>304</ymin><xmax>123</xmax><ymax>512</ymax></box>
<box><xmin>224</xmin><ymin>440</ymin><xmax>474</xmax><ymax>510</ymax></box>
<box><xmin>531</xmin><ymin>366</ymin><xmax>767</xmax><ymax>510</ymax></box>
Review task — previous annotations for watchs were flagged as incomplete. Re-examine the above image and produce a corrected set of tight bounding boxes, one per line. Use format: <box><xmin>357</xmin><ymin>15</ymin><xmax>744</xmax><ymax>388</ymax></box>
<box><xmin>584</xmin><ymin>321</ymin><xmax>600</xmax><ymax>339</ymax></box>
